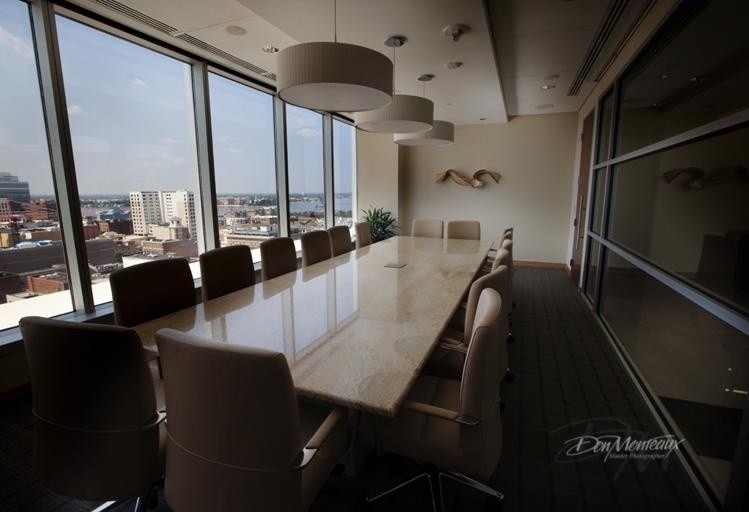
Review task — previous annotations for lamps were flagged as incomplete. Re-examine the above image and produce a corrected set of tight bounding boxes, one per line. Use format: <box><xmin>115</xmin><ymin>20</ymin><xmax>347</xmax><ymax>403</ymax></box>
<box><xmin>390</xmin><ymin>74</ymin><xmax>455</xmax><ymax>146</ymax></box>
<box><xmin>354</xmin><ymin>38</ymin><xmax>433</xmax><ymax>134</ymax></box>
<box><xmin>277</xmin><ymin>0</ymin><xmax>393</xmax><ymax>113</ymax></box>
